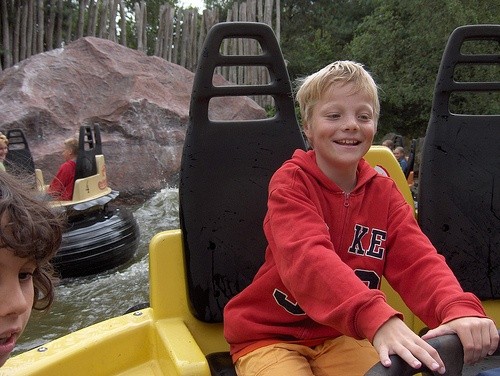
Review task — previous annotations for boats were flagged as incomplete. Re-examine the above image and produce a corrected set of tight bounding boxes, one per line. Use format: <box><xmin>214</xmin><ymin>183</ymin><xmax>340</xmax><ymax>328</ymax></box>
<box><xmin>0</xmin><ymin>122</ymin><xmax>141</xmax><ymax>279</ymax></box>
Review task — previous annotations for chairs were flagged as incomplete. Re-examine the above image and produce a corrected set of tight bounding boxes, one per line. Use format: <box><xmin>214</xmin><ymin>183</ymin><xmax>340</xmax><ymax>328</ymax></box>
<box><xmin>0</xmin><ymin>23</ymin><xmax>500</xmax><ymax>376</ymax></box>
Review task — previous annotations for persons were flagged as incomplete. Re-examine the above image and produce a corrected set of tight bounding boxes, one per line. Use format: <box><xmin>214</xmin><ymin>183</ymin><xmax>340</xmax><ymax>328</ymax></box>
<box><xmin>381</xmin><ymin>138</ymin><xmax>420</xmax><ymax>187</ymax></box>
<box><xmin>223</xmin><ymin>59</ymin><xmax>500</xmax><ymax>376</ymax></box>
<box><xmin>0</xmin><ymin>131</ymin><xmax>8</xmax><ymax>174</ymax></box>
<box><xmin>32</xmin><ymin>137</ymin><xmax>81</xmax><ymax>202</ymax></box>
<box><xmin>0</xmin><ymin>167</ymin><xmax>67</xmax><ymax>366</ymax></box>
